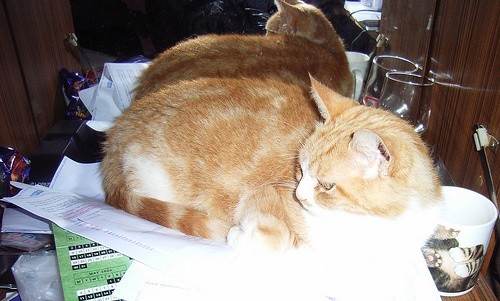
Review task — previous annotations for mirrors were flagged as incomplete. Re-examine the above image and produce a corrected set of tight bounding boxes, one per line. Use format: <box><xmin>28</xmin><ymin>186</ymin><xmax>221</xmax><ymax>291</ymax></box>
<box><xmin>2</xmin><ymin>0</ymin><xmax>440</xmax><ymax>162</ymax></box>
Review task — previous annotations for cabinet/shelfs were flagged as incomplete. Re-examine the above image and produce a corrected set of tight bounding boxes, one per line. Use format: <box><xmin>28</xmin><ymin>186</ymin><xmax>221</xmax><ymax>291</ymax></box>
<box><xmin>418</xmin><ymin>0</ymin><xmax>500</xmax><ymax>278</ymax></box>
<box><xmin>376</xmin><ymin>0</ymin><xmax>438</xmax><ymax>122</ymax></box>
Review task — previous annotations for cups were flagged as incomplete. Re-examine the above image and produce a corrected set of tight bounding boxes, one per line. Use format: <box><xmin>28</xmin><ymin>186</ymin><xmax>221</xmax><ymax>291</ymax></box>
<box><xmin>362</xmin><ymin>55</ymin><xmax>418</xmax><ymax>108</ymax></box>
<box><xmin>422</xmin><ymin>186</ymin><xmax>498</xmax><ymax>296</ymax></box>
<box><xmin>379</xmin><ymin>72</ymin><xmax>434</xmax><ymax>134</ymax></box>
<box><xmin>345</xmin><ymin>51</ymin><xmax>370</xmax><ymax>101</ymax></box>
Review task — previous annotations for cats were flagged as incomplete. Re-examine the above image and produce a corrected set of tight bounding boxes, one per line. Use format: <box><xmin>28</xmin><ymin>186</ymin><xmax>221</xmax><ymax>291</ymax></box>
<box><xmin>94</xmin><ymin>69</ymin><xmax>445</xmax><ymax>255</ymax></box>
<box><xmin>128</xmin><ymin>1</ymin><xmax>354</xmax><ymax>104</ymax></box>
